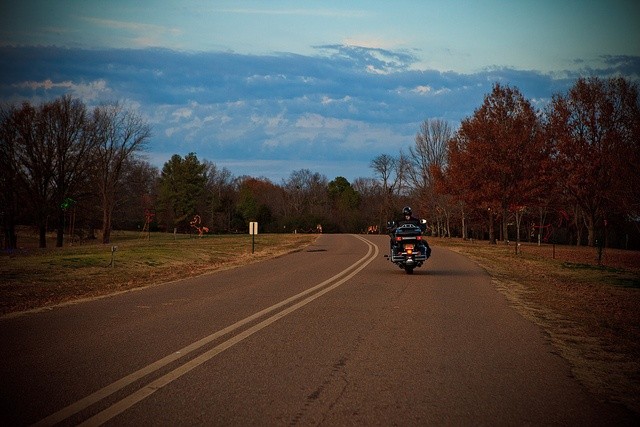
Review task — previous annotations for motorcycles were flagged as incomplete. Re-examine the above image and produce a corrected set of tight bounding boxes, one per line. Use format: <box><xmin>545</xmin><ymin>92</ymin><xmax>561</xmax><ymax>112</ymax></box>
<box><xmin>383</xmin><ymin>217</ymin><xmax>427</xmax><ymax>274</ymax></box>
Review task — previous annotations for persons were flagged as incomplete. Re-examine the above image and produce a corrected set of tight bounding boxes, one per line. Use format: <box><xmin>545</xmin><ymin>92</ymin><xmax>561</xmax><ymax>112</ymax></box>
<box><xmin>389</xmin><ymin>206</ymin><xmax>432</xmax><ymax>257</ymax></box>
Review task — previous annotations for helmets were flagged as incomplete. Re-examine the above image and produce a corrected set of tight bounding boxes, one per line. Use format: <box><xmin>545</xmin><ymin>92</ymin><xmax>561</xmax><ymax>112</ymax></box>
<box><xmin>402</xmin><ymin>206</ymin><xmax>412</xmax><ymax>215</ymax></box>
